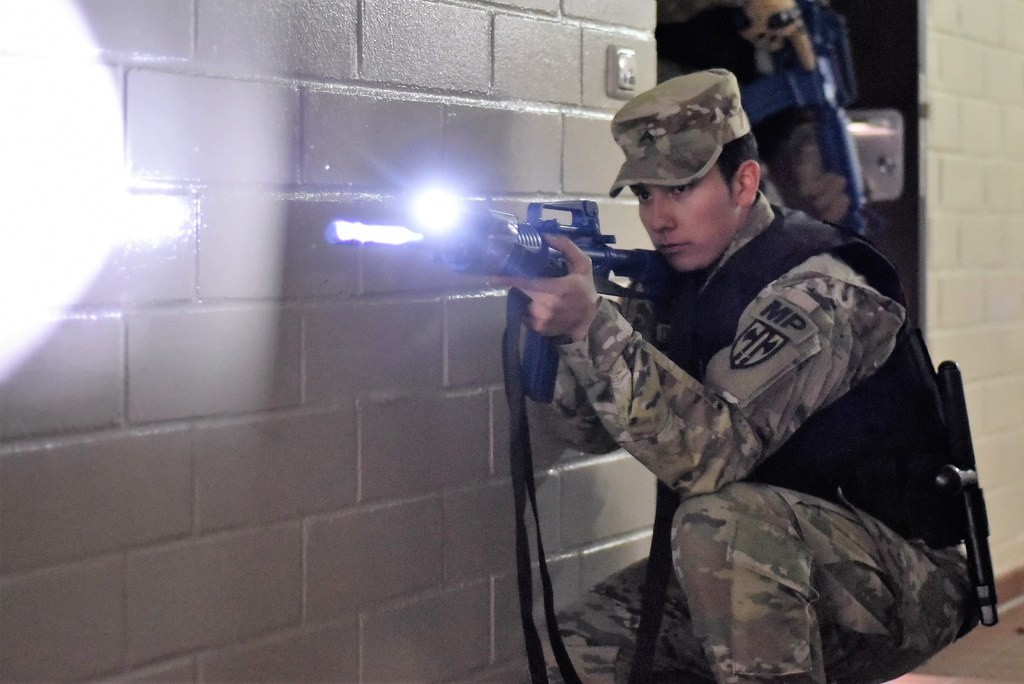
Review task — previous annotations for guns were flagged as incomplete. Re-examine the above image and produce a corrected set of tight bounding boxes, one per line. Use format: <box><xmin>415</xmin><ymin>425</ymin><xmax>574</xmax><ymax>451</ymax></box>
<box><xmin>324</xmin><ymin>199</ymin><xmax>674</xmax><ymax>404</ymax></box>
<box><xmin>743</xmin><ymin>0</ymin><xmax>869</xmax><ymax>236</ymax></box>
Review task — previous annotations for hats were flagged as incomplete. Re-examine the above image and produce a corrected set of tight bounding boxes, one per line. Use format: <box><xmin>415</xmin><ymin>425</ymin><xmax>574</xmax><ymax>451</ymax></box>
<box><xmin>609</xmin><ymin>69</ymin><xmax>751</xmax><ymax>199</ymax></box>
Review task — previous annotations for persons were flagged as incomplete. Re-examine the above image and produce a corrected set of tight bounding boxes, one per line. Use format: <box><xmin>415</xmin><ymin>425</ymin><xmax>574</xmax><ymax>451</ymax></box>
<box><xmin>659</xmin><ymin>2</ymin><xmax>873</xmax><ymax>229</ymax></box>
<box><xmin>490</xmin><ymin>68</ymin><xmax>985</xmax><ymax>684</ymax></box>
